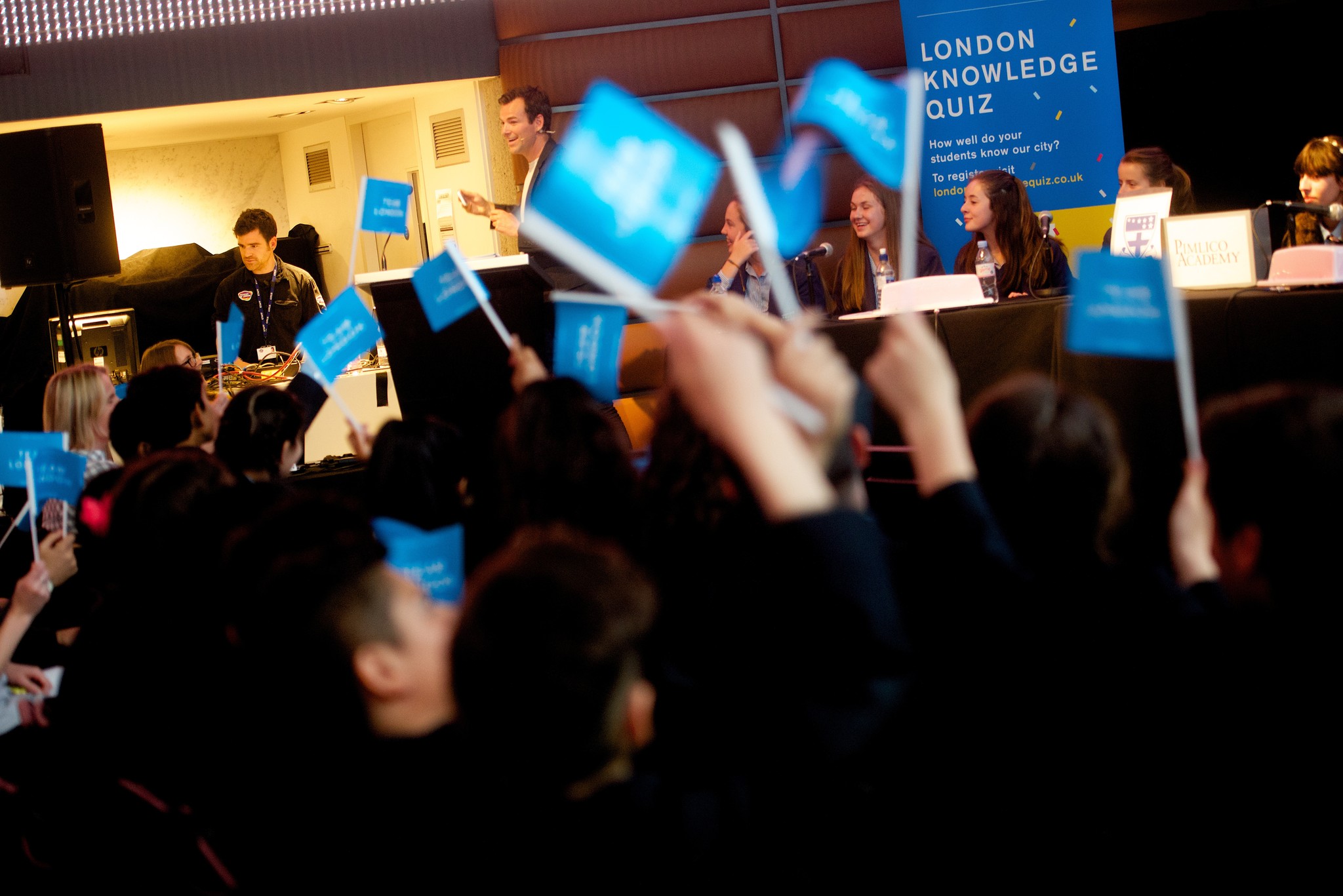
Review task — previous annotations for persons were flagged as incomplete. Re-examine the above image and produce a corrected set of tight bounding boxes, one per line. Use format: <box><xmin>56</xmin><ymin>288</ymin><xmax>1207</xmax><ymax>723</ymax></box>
<box><xmin>0</xmin><ymin>281</ymin><xmax>1342</xmax><ymax>896</ymax></box>
<box><xmin>952</xmin><ymin>170</ymin><xmax>1073</xmax><ymax>299</ymax></box>
<box><xmin>1270</xmin><ymin>133</ymin><xmax>1343</xmax><ymax>248</ymax></box>
<box><xmin>209</xmin><ymin>209</ymin><xmax>328</xmax><ymax>366</ymax></box>
<box><xmin>702</xmin><ymin>195</ymin><xmax>826</xmax><ymax>320</ymax></box>
<box><xmin>458</xmin><ymin>85</ymin><xmax>596</xmax><ymax>292</ymax></box>
<box><xmin>1100</xmin><ymin>144</ymin><xmax>1193</xmax><ymax>257</ymax></box>
<box><xmin>824</xmin><ymin>176</ymin><xmax>944</xmax><ymax>316</ymax></box>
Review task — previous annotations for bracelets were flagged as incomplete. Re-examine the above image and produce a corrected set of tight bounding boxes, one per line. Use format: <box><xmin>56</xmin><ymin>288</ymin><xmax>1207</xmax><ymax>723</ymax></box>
<box><xmin>726</xmin><ymin>258</ymin><xmax>745</xmax><ymax>291</ymax></box>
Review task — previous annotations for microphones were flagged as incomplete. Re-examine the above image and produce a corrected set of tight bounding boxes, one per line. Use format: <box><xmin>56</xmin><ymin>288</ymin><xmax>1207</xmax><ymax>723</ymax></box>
<box><xmin>795</xmin><ymin>243</ymin><xmax>833</xmax><ymax>262</ymax></box>
<box><xmin>1264</xmin><ymin>200</ymin><xmax>1343</xmax><ymax>220</ymax></box>
<box><xmin>1038</xmin><ymin>211</ymin><xmax>1054</xmax><ymax>239</ymax></box>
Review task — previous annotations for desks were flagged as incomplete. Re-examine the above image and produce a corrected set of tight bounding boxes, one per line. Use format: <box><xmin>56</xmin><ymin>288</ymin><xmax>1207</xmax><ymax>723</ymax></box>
<box><xmin>805</xmin><ymin>283</ymin><xmax>1343</xmax><ymax>481</ymax></box>
<box><xmin>206</xmin><ymin>366</ymin><xmax>404</xmax><ymax>469</ymax></box>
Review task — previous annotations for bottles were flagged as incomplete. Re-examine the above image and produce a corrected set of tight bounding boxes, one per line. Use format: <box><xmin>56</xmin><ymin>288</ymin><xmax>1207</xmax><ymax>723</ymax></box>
<box><xmin>974</xmin><ymin>240</ymin><xmax>999</xmax><ymax>304</ymax></box>
<box><xmin>876</xmin><ymin>247</ymin><xmax>895</xmax><ymax>311</ymax></box>
<box><xmin>710</xmin><ymin>274</ymin><xmax>726</xmax><ymax>294</ymax></box>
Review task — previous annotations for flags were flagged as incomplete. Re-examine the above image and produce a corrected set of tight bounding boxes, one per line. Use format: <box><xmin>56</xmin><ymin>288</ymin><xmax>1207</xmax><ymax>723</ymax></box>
<box><xmin>24</xmin><ymin>447</ymin><xmax>88</xmax><ymax>516</ymax></box>
<box><xmin>0</xmin><ymin>431</ymin><xmax>71</xmax><ymax>489</ymax></box>
<box><xmin>359</xmin><ymin>178</ymin><xmax>410</xmax><ymax>235</ymax></box>
<box><xmin>297</xmin><ymin>285</ymin><xmax>382</xmax><ymax>385</ymax></box>
<box><xmin>216</xmin><ymin>304</ymin><xmax>244</xmax><ymax>368</ymax></box>
<box><xmin>515</xmin><ymin>76</ymin><xmax>726</xmax><ymax>313</ymax></box>
<box><xmin>412</xmin><ymin>241</ymin><xmax>490</xmax><ymax>332</ymax></box>
<box><xmin>753</xmin><ymin>133</ymin><xmax>832</xmax><ymax>260</ymax></box>
<box><xmin>785</xmin><ymin>52</ymin><xmax>902</xmax><ymax>188</ymax></box>
<box><xmin>547</xmin><ymin>290</ymin><xmax>628</xmax><ymax>403</ymax></box>
<box><xmin>373</xmin><ymin>519</ymin><xmax>464</xmax><ymax>607</ymax></box>
<box><xmin>1061</xmin><ymin>253</ymin><xmax>1178</xmax><ymax>360</ymax></box>
